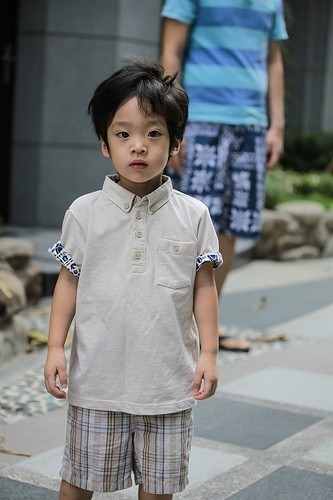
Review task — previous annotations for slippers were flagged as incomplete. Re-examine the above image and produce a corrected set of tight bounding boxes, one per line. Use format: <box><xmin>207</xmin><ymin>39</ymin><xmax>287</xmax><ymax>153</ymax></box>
<box><xmin>217</xmin><ymin>335</ymin><xmax>250</xmax><ymax>353</ymax></box>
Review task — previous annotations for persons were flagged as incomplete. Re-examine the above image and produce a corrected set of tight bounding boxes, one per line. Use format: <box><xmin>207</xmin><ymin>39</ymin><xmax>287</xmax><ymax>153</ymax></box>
<box><xmin>43</xmin><ymin>60</ymin><xmax>224</xmax><ymax>496</ymax></box>
<box><xmin>160</xmin><ymin>0</ymin><xmax>289</xmax><ymax>354</ymax></box>
